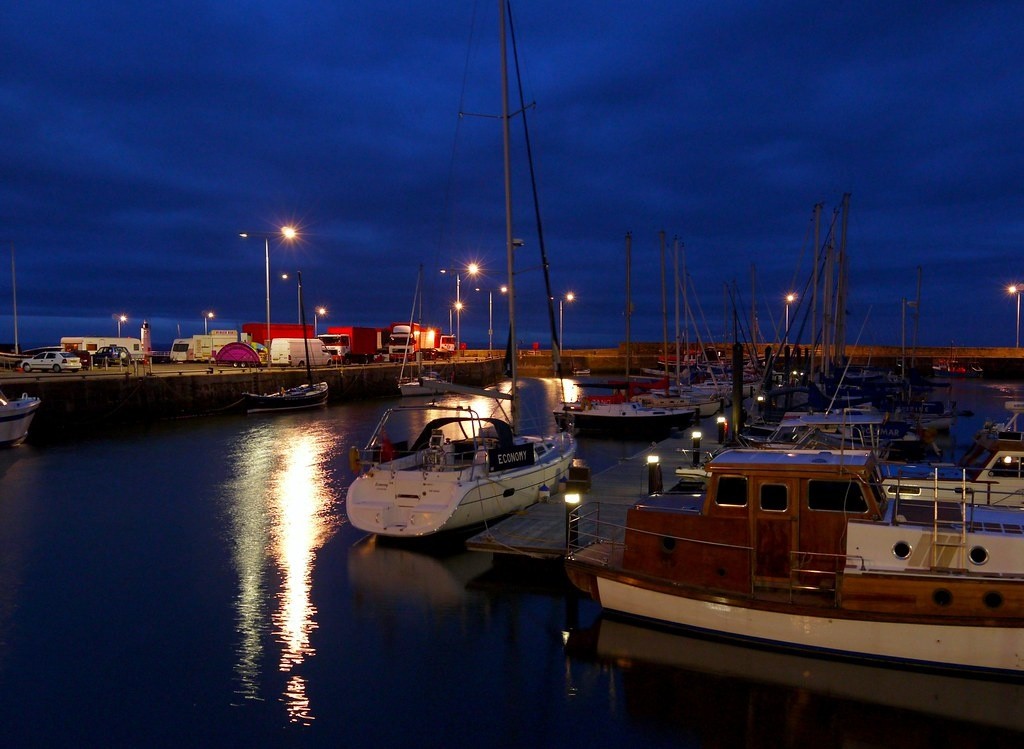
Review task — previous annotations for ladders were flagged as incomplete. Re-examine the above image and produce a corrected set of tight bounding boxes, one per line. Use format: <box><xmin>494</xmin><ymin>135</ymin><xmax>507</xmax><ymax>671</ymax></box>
<box><xmin>934</xmin><ymin>467</ymin><xmax>966</xmax><ymax>568</ymax></box>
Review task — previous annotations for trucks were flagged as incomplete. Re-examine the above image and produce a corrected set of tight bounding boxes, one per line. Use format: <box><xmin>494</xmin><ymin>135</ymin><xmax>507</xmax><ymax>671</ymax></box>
<box><xmin>385</xmin><ymin>322</ymin><xmax>456</xmax><ymax>363</ymax></box>
<box><xmin>317</xmin><ymin>325</ymin><xmax>391</xmax><ymax>365</ymax></box>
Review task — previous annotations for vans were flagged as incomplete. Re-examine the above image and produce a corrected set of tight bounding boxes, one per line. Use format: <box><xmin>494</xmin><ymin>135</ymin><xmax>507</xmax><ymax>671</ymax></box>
<box><xmin>270</xmin><ymin>337</ymin><xmax>333</xmax><ymax>369</ymax></box>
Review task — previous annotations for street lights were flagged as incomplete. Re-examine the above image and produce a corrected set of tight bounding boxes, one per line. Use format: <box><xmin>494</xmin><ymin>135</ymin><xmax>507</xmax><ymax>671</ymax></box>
<box><xmin>281</xmin><ymin>271</ymin><xmax>304</xmax><ymax>325</ymax></box>
<box><xmin>440</xmin><ymin>268</ymin><xmax>479</xmax><ymax>351</ymax></box>
<box><xmin>314</xmin><ymin>308</ymin><xmax>327</xmax><ymax>339</ymax></box>
<box><xmin>204</xmin><ymin>312</ymin><xmax>214</xmax><ymax>335</ymax></box>
<box><xmin>474</xmin><ymin>287</ymin><xmax>509</xmax><ymax>350</ymax></box>
<box><xmin>784</xmin><ymin>294</ymin><xmax>795</xmax><ymax>346</ymax></box>
<box><xmin>118</xmin><ymin>315</ymin><xmax>126</xmax><ymax>338</ymax></box>
<box><xmin>1008</xmin><ymin>284</ymin><xmax>1023</xmax><ymax>349</ymax></box>
<box><xmin>238</xmin><ymin>228</ymin><xmax>296</xmax><ymax>371</ymax></box>
<box><xmin>559</xmin><ymin>294</ymin><xmax>574</xmax><ymax>356</ymax></box>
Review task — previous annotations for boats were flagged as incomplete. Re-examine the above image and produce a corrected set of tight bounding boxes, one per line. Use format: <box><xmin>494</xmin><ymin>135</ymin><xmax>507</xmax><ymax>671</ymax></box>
<box><xmin>241</xmin><ymin>381</ymin><xmax>329</xmax><ymax>417</ymax></box>
<box><xmin>0</xmin><ymin>389</ymin><xmax>41</xmax><ymax>446</ymax></box>
<box><xmin>552</xmin><ymin>187</ymin><xmax>1024</xmax><ymax>734</ymax></box>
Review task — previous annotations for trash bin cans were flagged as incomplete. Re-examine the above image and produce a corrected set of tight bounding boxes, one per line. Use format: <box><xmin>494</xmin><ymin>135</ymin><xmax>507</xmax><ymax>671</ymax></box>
<box><xmin>416</xmin><ymin>351</ymin><xmax>422</xmax><ymax>362</ymax></box>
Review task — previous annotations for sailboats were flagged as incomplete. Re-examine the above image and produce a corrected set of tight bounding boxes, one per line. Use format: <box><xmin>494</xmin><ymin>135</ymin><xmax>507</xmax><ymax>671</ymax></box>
<box><xmin>344</xmin><ymin>0</ymin><xmax>576</xmax><ymax>541</ymax></box>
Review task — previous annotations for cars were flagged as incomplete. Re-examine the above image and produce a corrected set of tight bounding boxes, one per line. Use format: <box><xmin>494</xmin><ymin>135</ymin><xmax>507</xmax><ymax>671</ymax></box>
<box><xmin>18</xmin><ymin>350</ymin><xmax>83</xmax><ymax>373</ymax></box>
<box><xmin>70</xmin><ymin>349</ymin><xmax>91</xmax><ymax>371</ymax></box>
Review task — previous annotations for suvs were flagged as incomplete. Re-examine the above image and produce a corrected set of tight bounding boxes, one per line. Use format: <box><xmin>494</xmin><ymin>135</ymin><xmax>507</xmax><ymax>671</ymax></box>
<box><xmin>91</xmin><ymin>346</ymin><xmax>132</xmax><ymax>367</ymax></box>
